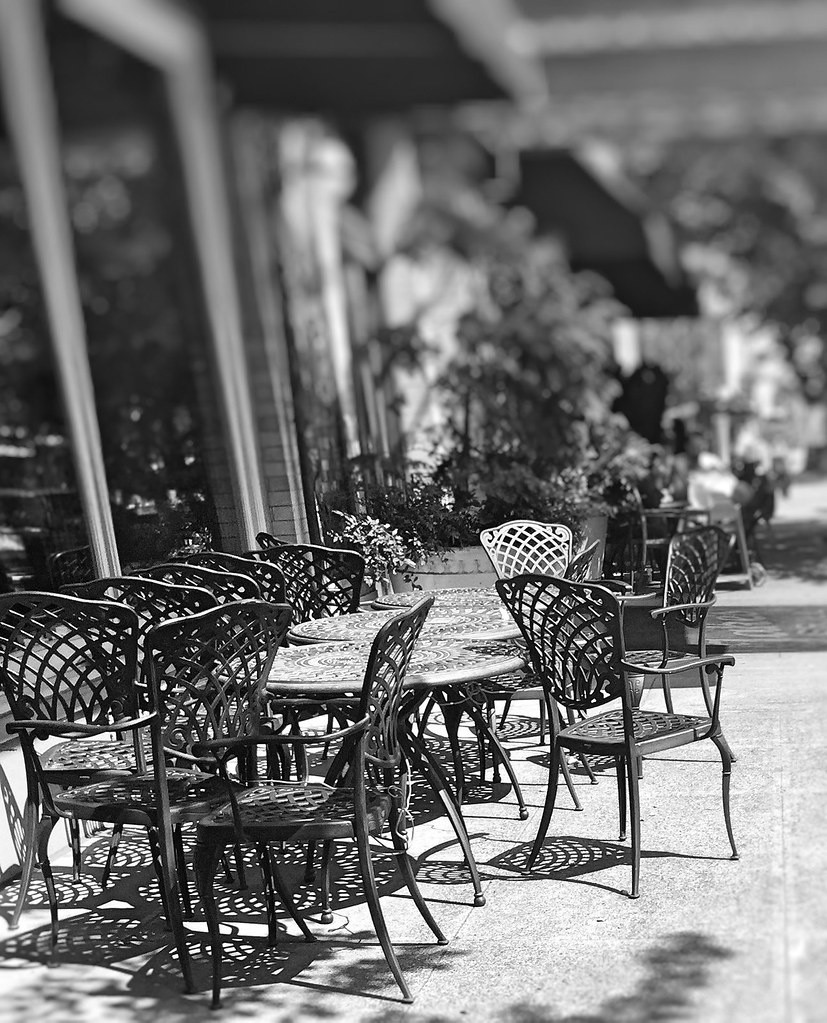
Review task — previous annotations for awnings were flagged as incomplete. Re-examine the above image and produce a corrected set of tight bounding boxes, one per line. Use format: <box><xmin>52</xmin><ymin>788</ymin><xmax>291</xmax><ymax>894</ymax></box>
<box><xmin>176</xmin><ymin>1</ymin><xmax>534</xmax><ymax>110</ymax></box>
<box><xmin>471</xmin><ymin>129</ymin><xmax>703</xmax><ymax>320</ymax></box>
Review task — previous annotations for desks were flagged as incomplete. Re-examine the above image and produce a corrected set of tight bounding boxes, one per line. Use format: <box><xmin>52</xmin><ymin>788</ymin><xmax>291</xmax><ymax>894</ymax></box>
<box><xmin>371</xmin><ymin>587</ymin><xmax>568</xmax><ymax>783</ymax></box>
<box><xmin>212</xmin><ymin>639</ymin><xmax>530</xmax><ymax>923</ymax></box>
<box><xmin>286</xmin><ymin>609</ymin><xmax>549</xmax><ymax>819</ymax></box>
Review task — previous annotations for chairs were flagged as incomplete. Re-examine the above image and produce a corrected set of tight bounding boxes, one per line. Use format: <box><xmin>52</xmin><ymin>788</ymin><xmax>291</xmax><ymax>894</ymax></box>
<box><xmin>0</xmin><ymin>518</ymin><xmax>738</xmax><ymax>1004</ymax></box>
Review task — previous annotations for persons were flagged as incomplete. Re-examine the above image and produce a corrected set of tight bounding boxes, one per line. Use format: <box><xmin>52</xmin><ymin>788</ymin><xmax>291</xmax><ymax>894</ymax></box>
<box><xmin>677</xmin><ymin>451</ymin><xmax>759</xmax><ymax>535</ymax></box>
<box><xmin>638</xmin><ymin>452</ymin><xmax>668</xmax><ymax>507</ymax></box>
